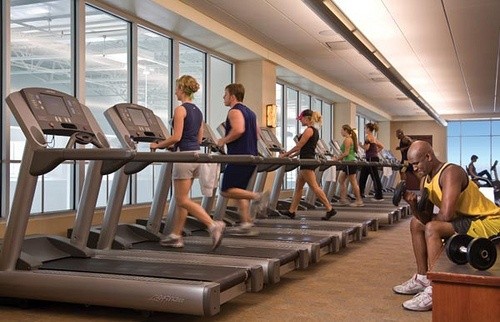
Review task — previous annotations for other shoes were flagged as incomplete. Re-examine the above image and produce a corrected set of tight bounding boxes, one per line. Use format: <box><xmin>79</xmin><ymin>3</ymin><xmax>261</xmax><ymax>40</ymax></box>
<box><xmin>257</xmin><ymin>190</ymin><xmax>271</xmax><ymax>213</ymax></box>
<box><xmin>229</xmin><ymin>226</ymin><xmax>259</xmax><ymax>236</ymax></box>
<box><xmin>349</xmin><ymin>201</ymin><xmax>364</xmax><ymax>206</ymax></box>
<box><xmin>371</xmin><ymin>198</ymin><xmax>385</xmax><ymax>201</ymax></box>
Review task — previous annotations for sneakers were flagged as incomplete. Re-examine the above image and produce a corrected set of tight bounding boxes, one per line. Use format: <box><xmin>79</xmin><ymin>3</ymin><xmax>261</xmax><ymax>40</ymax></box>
<box><xmin>278</xmin><ymin>210</ymin><xmax>295</xmax><ymax>219</ymax></box>
<box><xmin>321</xmin><ymin>208</ymin><xmax>337</xmax><ymax>220</ymax></box>
<box><xmin>159</xmin><ymin>236</ymin><xmax>185</xmax><ymax>247</ymax></box>
<box><xmin>402</xmin><ymin>286</ymin><xmax>432</xmax><ymax>311</ymax></box>
<box><xmin>210</xmin><ymin>221</ymin><xmax>227</xmax><ymax>251</ymax></box>
<box><xmin>392</xmin><ymin>274</ymin><xmax>432</xmax><ymax>294</ymax></box>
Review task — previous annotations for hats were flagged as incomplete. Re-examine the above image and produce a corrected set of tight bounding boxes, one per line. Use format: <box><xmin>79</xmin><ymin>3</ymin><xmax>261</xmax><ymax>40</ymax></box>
<box><xmin>296</xmin><ymin>109</ymin><xmax>313</xmax><ymax>120</ymax></box>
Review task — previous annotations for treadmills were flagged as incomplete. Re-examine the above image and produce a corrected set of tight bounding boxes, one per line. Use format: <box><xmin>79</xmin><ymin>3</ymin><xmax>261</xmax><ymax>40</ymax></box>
<box><xmin>0</xmin><ymin>85</ymin><xmax>411</xmax><ymax>322</ymax></box>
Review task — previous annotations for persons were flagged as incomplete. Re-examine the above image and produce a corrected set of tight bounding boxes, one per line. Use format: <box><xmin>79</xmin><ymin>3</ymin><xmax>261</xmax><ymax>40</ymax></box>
<box><xmin>392</xmin><ymin>141</ymin><xmax>500</xmax><ymax>311</ymax></box>
<box><xmin>333</xmin><ymin>125</ymin><xmax>364</xmax><ymax>206</ymax></box>
<box><xmin>396</xmin><ymin>128</ymin><xmax>422</xmax><ymax>184</ymax></box>
<box><xmin>278</xmin><ymin>110</ymin><xmax>337</xmax><ymax>221</ymax></box>
<box><xmin>469</xmin><ymin>154</ymin><xmax>493</xmax><ymax>185</ymax></box>
<box><xmin>358</xmin><ymin>121</ymin><xmax>384</xmax><ymax>202</ymax></box>
<box><xmin>214</xmin><ymin>84</ymin><xmax>270</xmax><ymax>237</ymax></box>
<box><xmin>149</xmin><ymin>76</ymin><xmax>226</xmax><ymax>252</ymax></box>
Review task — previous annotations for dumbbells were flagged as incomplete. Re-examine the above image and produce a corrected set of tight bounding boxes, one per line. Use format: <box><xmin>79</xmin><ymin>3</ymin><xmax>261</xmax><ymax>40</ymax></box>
<box><xmin>446</xmin><ymin>233</ymin><xmax>497</xmax><ymax>270</ymax></box>
<box><xmin>392</xmin><ymin>179</ymin><xmax>430</xmax><ymax>211</ymax></box>
